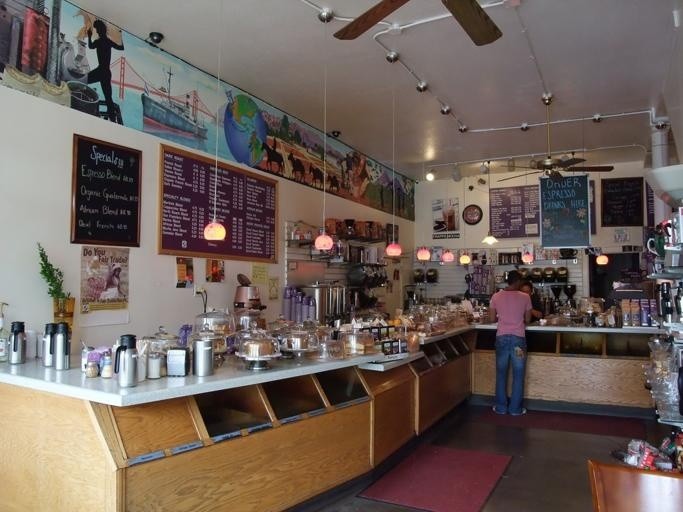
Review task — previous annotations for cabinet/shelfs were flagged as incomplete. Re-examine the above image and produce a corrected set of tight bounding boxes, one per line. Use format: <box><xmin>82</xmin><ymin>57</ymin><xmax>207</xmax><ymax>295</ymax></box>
<box><xmin>1</xmin><ymin>321</ymin><xmax>470</xmax><ymax>512</ymax></box>
<box><xmin>472</xmin><ymin>324</ymin><xmax>675</xmax><ymax>420</ymax></box>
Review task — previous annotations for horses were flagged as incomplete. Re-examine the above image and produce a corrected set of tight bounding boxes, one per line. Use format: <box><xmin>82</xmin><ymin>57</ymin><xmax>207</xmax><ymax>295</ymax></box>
<box><xmin>260</xmin><ymin>140</ymin><xmax>285</xmax><ymax>177</ymax></box>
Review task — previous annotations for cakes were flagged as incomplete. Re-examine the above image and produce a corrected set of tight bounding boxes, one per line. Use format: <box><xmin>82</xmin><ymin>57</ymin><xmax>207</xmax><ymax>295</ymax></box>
<box><xmin>581</xmin><ymin>302</ymin><xmax>602</xmax><ymax>314</ymax></box>
<box><xmin>212</xmin><ymin>318</ymin><xmax>379</xmax><ymax>360</ymax></box>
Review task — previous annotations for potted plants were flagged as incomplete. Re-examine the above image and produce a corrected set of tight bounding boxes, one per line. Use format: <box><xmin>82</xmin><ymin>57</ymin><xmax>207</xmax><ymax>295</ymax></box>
<box><xmin>36</xmin><ymin>242</ymin><xmax>75</xmax><ymax>325</ymax></box>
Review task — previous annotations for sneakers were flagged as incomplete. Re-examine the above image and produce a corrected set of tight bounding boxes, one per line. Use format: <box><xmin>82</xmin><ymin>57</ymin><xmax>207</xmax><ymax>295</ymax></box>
<box><xmin>512</xmin><ymin>408</ymin><xmax>528</xmax><ymax>417</ymax></box>
<box><xmin>493</xmin><ymin>406</ymin><xmax>508</xmax><ymax>416</ymax></box>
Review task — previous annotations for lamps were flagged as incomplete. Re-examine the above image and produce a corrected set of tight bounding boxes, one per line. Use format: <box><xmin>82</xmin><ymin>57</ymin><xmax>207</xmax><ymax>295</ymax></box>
<box><xmin>416</xmin><ymin>81</ymin><xmax>431</xmax><ymax>262</ymax></box>
<box><xmin>385</xmin><ymin>49</ymin><xmax>402</xmax><ymax>257</ymax></box>
<box><xmin>458</xmin><ymin>124</ymin><xmax>470</xmax><ymax>264</ymax></box>
<box><xmin>481</xmin><ymin>159</ymin><xmax>498</xmax><ymax>245</ymax></box>
<box><xmin>204</xmin><ymin>1</ymin><xmax>226</xmax><ymax>241</ymax></box>
<box><xmin>592</xmin><ymin>112</ymin><xmax>609</xmax><ymax>264</ymax></box>
<box><xmin>423</xmin><ymin>168</ymin><xmax>437</xmax><ymax>183</ymax></box>
<box><xmin>439</xmin><ymin>104</ymin><xmax>455</xmax><ymax>263</ymax></box>
<box><xmin>313</xmin><ymin>7</ymin><xmax>334</xmax><ymax>250</ymax></box>
<box><xmin>521</xmin><ymin>155</ymin><xmax>535</xmax><ymax>264</ymax></box>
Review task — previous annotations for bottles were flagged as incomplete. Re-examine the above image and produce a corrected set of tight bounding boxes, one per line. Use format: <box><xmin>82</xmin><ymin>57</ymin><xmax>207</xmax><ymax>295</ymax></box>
<box><xmin>282</xmin><ymin>286</ymin><xmax>316</xmax><ymax>323</ymax></box>
<box><xmin>583</xmin><ymin>311</ymin><xmax>607</xmax><ymax>327</ymax></box>
<box><xmin>147</xmin><ymin>353</ymin><xmax>161</xmax><ymax>379</ymax></box>
<box><xmin>80</xmin><ymin>346</ymin><xmax>112</xmax><ymax>378</ymax></box>
<box><xmin>655</xmin><ymin>282</ymin><xmax>683</xmax><ymax>325</ymax></box>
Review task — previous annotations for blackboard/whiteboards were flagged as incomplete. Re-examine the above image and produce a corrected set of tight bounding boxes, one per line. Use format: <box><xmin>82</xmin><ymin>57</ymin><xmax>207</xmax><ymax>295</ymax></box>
<box><xmin>538</xmin><ymin>173</ymin><xmax>592</xmax><ymax>250</ymax></box>
<box><xmin>70</xmin><ymin>133</ymin><xmax>142</xmax><ymax>247</ymax></box>
<box><xmin>489</xmin><ymin>180</ymin><xmax>596</xmax><ymax>238</ymax></box>
<box><xmin>601</xmin><ymin>177</ymin><xmax>644</xmax><ymax>227</ymax></box>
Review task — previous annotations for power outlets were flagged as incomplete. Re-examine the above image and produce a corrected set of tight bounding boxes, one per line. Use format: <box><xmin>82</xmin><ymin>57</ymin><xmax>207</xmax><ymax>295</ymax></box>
<box><xmin>193</xmin><ymin>284</ymin><xmax>204</xmax><ymax>298</ymax></box>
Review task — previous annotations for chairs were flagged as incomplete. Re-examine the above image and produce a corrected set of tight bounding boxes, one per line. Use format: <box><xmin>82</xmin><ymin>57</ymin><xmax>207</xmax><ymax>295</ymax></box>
<box><xmin>587</xmin><ymin>458</ymin><xmax>681</xmax><ymax>511</ymax></box>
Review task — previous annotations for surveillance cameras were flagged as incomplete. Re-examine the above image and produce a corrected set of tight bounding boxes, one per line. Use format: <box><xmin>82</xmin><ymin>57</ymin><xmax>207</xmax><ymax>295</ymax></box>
<box><xmin>149</xmin><ymin>32</ymin><xmax>163</xmax><ymax>44</ymax></box>
<box><xmin>332</xmin><ymin>130</ymin><xmax>341</xmax><ymax>137</ymax></box>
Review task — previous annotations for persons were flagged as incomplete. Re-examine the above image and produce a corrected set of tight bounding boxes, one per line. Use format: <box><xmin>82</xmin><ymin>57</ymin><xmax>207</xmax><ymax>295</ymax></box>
<box><xmin>86</xmin><ymin>19</ymin><xmax>124</xmax><ymax>124</ymax></box>
<box><xmin>485</xmin><ymin>268</ymin><xmax>532</xmax><ymax>416</ymax></box>
<box><xmin>103</xmin><ymin>261</ymin><xmax>127</xmax><ymax>299</ymax></box>
<box><xmin>519</xmin><ymin>282</ymin><xmax>543</xmax><ymax>324</ymax></box>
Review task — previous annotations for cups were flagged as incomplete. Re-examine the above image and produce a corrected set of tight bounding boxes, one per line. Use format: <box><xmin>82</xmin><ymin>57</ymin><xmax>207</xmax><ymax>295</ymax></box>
<box><xmin>642</xmin><ymin>341</ymin><xmax>680</xmax><ymax>422</ymax></box>
<box><xmin>8</xmin><ymin>321</ymin><xmax>26</xmax><ymax>365</ymax></box>
<box><xmin>114</xmin><ymin>334</ymin><xmax>138</xmax><ymax>387</ymax></box>
<box><xmin>41</xmin><ymin>322</ymin><xmax>70</xmax><ymax>371</ymax></box>
<box><xmin>193</xmin><ymin>339</ymin><xmax>214</xmax><ymax>377</ymax></box>
